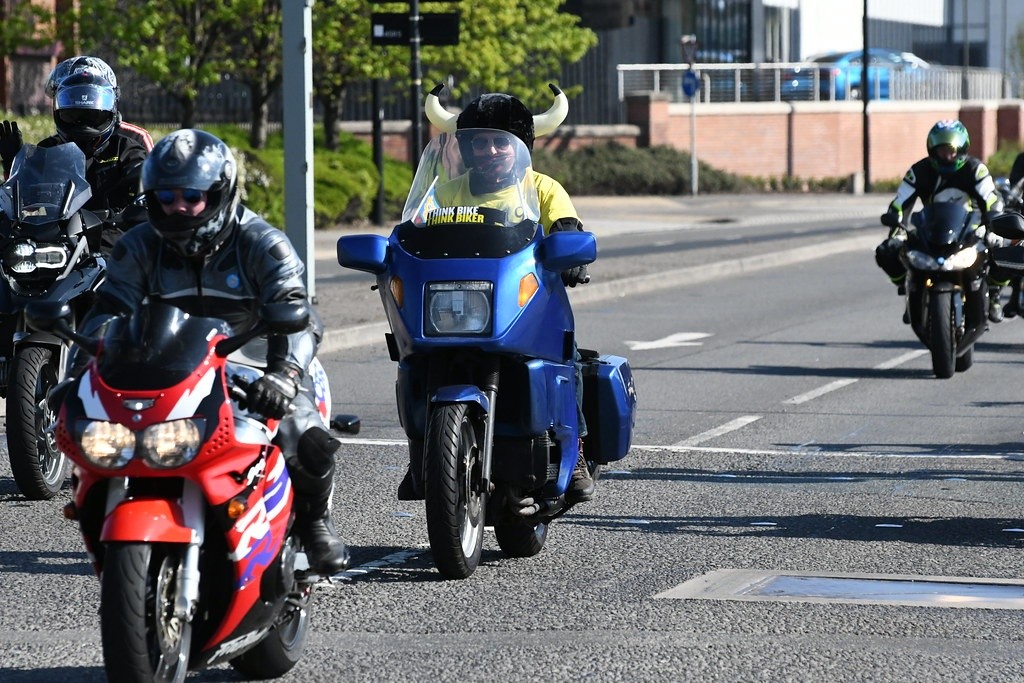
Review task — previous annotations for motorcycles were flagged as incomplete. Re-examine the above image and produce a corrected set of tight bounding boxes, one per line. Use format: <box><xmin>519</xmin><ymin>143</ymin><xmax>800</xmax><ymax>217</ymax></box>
<box><xmin>335</xmin><ymin>126</ymin><xmax>639</xmax><ymax>579</ymax></box>
<box><xmin>23</xmin><ymin>290</ymin><xmax>362</xmax><ymax>683</ymax></box>
<box><xmin>881</xmin><ymin>176</ymin><xmax>1024</xmax><ymax>379</ymax></box>
<box><xmin>0</xmin><ymin>141</ymin><xmax>106</xmax><ymax>499</ymax></box>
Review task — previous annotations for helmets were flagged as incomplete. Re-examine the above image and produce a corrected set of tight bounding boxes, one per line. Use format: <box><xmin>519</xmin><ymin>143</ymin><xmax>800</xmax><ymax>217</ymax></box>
<box><xmin>44</xmin><ymin>57</ymin><xmax>119</xmax><ymax>152</ymax></box>
<box><xmin>927</xmin><ymin>119</ymin><xmax>970</xmax><ymax>172</ymax></box>
<box><xmin>139</xmin><ymin>128</ymin><xmax>237</xmax><ymax>259</ymax></box>
<box><xmin>426</xmin><ymin>82</ymin><xmax>570</xmax><ymax>168</ymax></box>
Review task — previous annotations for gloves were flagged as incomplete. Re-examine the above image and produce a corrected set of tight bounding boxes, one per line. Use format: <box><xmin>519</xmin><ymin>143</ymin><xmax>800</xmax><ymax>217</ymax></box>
<box><xmin>0</xmin><ymin>119</ymin><xmax>24</xmax><ymax>178</ymax></box>
<box><xmin>888</xmin><ymin>230</ymin><xmax>907</xmax><ymax>247</ymax></box>
<box><xmin>983</xmin><ymin>230</ymin><xmax>1004</xmax><ymax>250</ymax></box>
<box><xmin>238</xmin><ymin>362</ymin><xmax>303</xmax><ymax>420</ymax></box>
<box><xmin>550</xmin><ymin>215</ymin><xmax>590</xmax><ymax>287</ymax></box>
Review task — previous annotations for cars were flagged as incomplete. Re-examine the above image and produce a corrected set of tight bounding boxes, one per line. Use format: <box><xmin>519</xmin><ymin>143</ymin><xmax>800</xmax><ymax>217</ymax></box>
<box><xmin>781</xmin><ymin>49</ymin><xmax>932</xmax><ymax>100</ymax></box>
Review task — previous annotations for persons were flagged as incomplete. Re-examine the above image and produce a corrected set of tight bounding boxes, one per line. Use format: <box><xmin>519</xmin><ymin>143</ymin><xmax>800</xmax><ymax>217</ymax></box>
<box><xmin>0</xmin><ymin>55</ymin><xmax>155</xmax><ymax>329</ymax></box>
<box><xmin>76</xmin><ymin>129</ymin><xmax>351</xmax><ymax>576</ymax></box>
<box><xmin>875</xmin><ymin>120</ymin><xmax>1003</xmax><ymax>324</ymax></box>
<box><xmin>398</xmin><ymin>83</ymin><xmax>594</xmax><ymax>503</ymax></box>
<box><xmin>1002</xmin><ymin>152</ymin><xmax>1024</xmax><ymax>318</ymax></box>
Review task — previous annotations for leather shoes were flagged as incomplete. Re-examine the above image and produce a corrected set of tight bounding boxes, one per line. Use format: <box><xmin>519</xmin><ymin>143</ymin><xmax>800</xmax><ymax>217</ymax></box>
<box><xmin>302</xmin><ymin>516</ymin><xmax>343</xmax><ymax>574</ymax></box>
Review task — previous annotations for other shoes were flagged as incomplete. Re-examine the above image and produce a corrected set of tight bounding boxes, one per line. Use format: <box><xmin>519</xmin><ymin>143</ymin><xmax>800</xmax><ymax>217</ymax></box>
<box><xmin>987</xmin><ymin>288</ymin><xmax>1004</xmax><ymax>321</ymax></box>
<box><xmin>898</xmin><ymin>288</ymin><xmax>911</xmax><ymax>324</ymax></box>
<box><xmin>398</xmin><ymin>467</ymin><xmax>422</xmax><ymax>499</ymax></box>
<box><xmin>566</xmin><ymin>449</ymin><xmax>593</xmax><ymax>501</ymax></box>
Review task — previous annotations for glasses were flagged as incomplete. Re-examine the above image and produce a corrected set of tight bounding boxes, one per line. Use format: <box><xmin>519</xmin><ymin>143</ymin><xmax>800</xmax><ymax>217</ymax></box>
<box><xmin>469</xmin><ymin>135</ymin><xmax>511</xmax><ymax>150</ymax></box>
<box><xmin>152</xmin><ymin>190</ymin><xmax>205</xmax><ymax>206</ymax></box>
